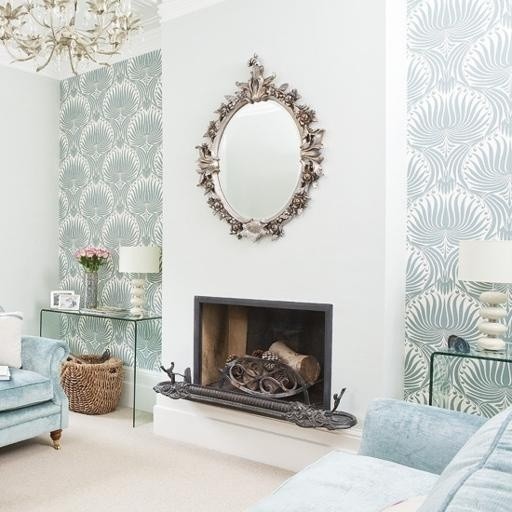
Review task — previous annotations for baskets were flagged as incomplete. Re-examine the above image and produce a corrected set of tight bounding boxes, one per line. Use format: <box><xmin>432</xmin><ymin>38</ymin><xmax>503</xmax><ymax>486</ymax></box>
<box><xmin>61</xmin><ymin>355</ymin><xmax>122</xmax><ymax>415</ymax></box>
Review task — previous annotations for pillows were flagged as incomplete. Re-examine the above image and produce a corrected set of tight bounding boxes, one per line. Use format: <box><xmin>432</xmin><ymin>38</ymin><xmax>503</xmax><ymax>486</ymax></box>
<box><xmin>0</xmin><ymin>312</ymin><xmax>23</xmax><ymax>369</ymax></box>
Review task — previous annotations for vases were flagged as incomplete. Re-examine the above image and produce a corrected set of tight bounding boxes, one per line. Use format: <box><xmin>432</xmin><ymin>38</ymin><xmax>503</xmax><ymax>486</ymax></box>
<box><xmin>86</xmin><ymin>271</ymin><xmax>98</xmax><ymax>309</ymax></box>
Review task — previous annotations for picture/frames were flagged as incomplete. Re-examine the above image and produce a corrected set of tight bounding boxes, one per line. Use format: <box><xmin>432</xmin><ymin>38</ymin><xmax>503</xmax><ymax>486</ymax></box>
<box><xmin>51</xmin><ymin>290</ymin><xmax>74</xmax><ymax>309</ymax></box>
<box><xmin>59</xmin><ymin>295</ymin><xmax>80</xmax><ymax>310</ymax></box>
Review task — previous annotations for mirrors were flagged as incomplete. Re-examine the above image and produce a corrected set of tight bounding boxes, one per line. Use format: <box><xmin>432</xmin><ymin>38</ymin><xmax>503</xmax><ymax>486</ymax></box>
<box><xmin>194</xmin><ymin>52</ymin><xmax>326</xmax><ymax>244</ymax></box>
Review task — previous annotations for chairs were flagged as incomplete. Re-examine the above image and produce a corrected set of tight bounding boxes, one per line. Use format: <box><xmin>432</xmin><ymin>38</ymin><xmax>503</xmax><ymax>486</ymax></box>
<box><xmin>0</xmin><ymin>306</ymin><xmax>70</xmax><ymax>450</ymax></box>
<box><xmin>249</xmin><ymin>397</ymin><xmax>511</xmax><ymax>512</ymax></box>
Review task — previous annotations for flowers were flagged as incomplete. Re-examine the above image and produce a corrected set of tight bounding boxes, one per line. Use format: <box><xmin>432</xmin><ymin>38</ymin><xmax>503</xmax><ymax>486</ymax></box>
<box><xmin>75</xmin><ymin>246</ymin><xmax>112</xmax><ymax>273</ymax></box>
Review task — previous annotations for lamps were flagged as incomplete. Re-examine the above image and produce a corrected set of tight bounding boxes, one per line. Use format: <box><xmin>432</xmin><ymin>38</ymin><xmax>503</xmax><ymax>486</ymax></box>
<box><xmin>0</xmin><ymin>1</ymin><xmax>142</xmax><ymax>77</ymax></box>
<box><xmin>119</xmin><ymin>247</ymin><xmax>159</xmax><ymax>315</ymax></box>
<box><xmin>458</xmin><ymin>240</ymin><xmax>512</xmax><ymax>352</ymax></box>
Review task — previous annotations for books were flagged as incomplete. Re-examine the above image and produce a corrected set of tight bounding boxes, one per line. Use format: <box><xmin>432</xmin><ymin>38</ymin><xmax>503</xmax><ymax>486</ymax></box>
<box><xmin>0</xmin><ymin>365</ymin><xmax>11</xmax><ymax>381</ymax></box>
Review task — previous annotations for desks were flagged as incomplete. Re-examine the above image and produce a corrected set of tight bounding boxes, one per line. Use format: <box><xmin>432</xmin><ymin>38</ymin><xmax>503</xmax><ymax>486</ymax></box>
<box><xmin>40</xmin><ymin>307</ymin><xmax>162</xmax><ymax>428</ymax></box>
<box><xmin>429</xmin><ymin>349</ymin><xmax>512</xmax><ymax>406</ymax></box>
